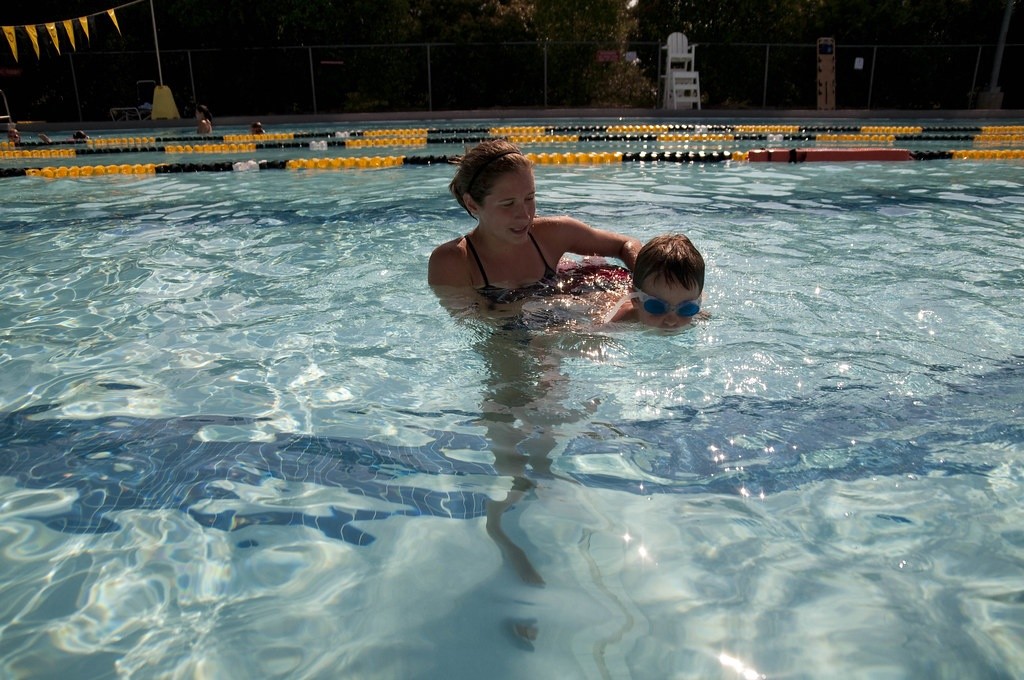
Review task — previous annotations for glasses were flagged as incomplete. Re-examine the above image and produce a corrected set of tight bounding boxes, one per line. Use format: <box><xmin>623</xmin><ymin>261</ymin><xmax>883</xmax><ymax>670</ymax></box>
<box><xmin>631</xmin><ymin>285</ymin><xmax>702</xmax><ymax>318</ymax></box>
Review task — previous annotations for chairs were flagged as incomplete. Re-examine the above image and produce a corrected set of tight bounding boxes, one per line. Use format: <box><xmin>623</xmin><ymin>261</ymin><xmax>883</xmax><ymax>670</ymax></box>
<box><xmin>661</xmin><ymin>32</ymin><xmax>699</xmax><ymax>75</ymax></box>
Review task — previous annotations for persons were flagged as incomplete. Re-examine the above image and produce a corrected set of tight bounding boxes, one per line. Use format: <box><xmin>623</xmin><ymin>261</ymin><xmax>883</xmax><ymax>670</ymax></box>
<box><xmin>251</xmin><ymin>122</ymin><xmax>264</xmax><ymax>134</ymax></box>
<box><xmin>8</xmin><ymin>129</ymin><xmax>20</xmax><ymax>142</ymax></box>
<box><xmin>196</xmin><ymin>105</ymin><xmax>213</xmax><ymax>134</ymax></box>
<box><xmin>612</xmin><ymin>234</ymin><xmax>706</xmax><ymax>329</ymax></box>
<box><xmin>429</xmin><ymin>141</ymin><xmax>639</xmax><ymax>289</ymax></box>
<box><xmin>39</xmin><ymin>131</ymin><xmax>89</xmax><ymax>143</ymax></box>
